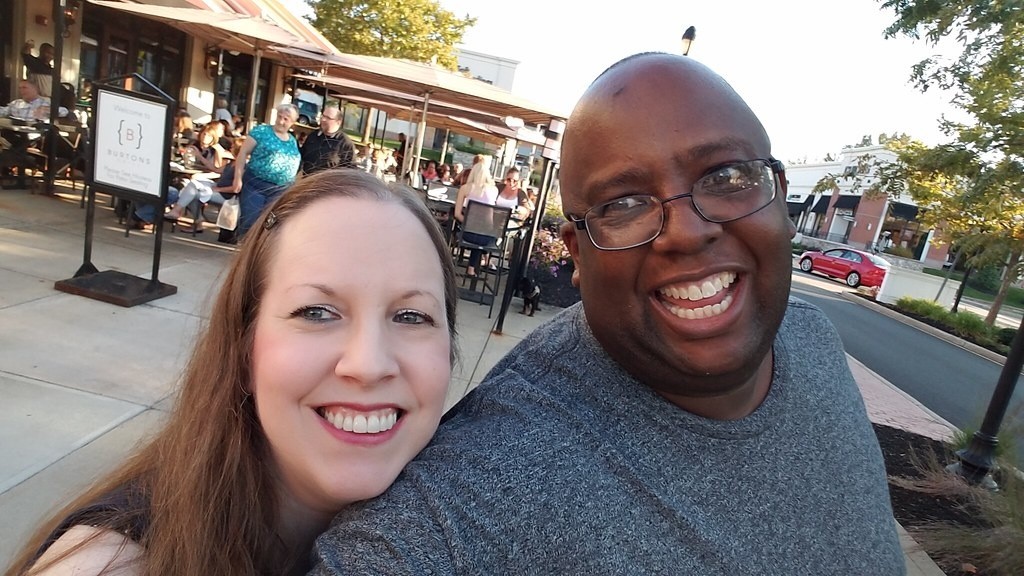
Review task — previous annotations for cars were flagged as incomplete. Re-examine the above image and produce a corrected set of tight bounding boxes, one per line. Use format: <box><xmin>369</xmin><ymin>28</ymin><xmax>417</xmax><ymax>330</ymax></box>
<box><xmin>799</xmin><ymin>247</ymin><xmax>892</xmax><ymax>288</ymax></box>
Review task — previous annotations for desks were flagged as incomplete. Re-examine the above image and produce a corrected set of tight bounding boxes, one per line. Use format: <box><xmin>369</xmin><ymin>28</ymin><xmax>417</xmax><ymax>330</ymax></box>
<box><xmin>152</xmin><ymin>166</ymin><xmax>222</xmax><ymax>234</ymax></box>
<box><xmin>425</xmin><ymin>180</ymin><xmax>524</xmax><ymax>297</ymax></box>
<box><xmin>0</xmin><ymin>114</ymin><xmax>77</xmax><ymax>194</ymax></box>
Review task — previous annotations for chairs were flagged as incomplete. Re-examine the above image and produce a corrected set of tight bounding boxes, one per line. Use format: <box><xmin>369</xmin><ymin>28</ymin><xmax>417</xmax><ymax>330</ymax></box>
<box><xmin>0</xmin><ymin>102</ymin><xmax>542</xmax><ymax>319</ymax></box>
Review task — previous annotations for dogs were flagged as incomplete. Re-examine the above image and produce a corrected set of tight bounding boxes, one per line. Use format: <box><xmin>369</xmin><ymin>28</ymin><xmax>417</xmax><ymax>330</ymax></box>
<box><xmin>514</xmin><ymin>276</ymin><xmax>542</xmax><ymax>317</ymax></box>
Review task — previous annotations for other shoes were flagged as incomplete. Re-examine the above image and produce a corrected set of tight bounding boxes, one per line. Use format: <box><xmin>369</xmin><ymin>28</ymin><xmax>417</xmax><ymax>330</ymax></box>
<box><xmin>129</xmin><ymin>216</ymin><xmax>154</xmax><ymax>229</ymax></box>
<box><xmin>466</xmin><ymin>272</ymin><xmax>477</xmax><ymax>277</ymax></box>
<box><xmin>480</xmin><ymin>259</ymin><xmax>496</xmax><ymax>270</ymax></box>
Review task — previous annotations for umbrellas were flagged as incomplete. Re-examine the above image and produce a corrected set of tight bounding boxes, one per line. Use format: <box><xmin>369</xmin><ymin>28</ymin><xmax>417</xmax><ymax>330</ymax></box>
<box><xmin>331</xmin><ymin>91</ymin><xmax>522</xmax><ymax>180</ymax></box>
<box><xmin>282</xmin><ymin>74</ymin><xmax>562</xmax><ymax>234</ymax></box>
<box><xmin>268</xmin><ymin>45</ymin><xmax>574</xmax><ymax>334</ymax></box>
<box><xmin>88</xmin><ymin>0</ymin><xmax>350</xmax><ymax>138</ymax></box>
<box><xmin>393</xmin><ymin>115</ymin><xmax>511</xmax><ymax>167</ymax></box>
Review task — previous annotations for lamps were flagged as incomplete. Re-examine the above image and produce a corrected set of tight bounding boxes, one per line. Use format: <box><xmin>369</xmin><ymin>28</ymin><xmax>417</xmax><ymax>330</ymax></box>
<box><xmin>204</xmin><ymin>44</ymin><xmax>218</xmax><ymax>80</ymax></box>
<box><xmin>62</xmin><ymin>0</ymin><xmax>79</xmax><ymax>38</ymax></box>
<box><xmin>281</xmin><ymin>74</ymin><xmax>294</xmax><ymax>97</ymax></box>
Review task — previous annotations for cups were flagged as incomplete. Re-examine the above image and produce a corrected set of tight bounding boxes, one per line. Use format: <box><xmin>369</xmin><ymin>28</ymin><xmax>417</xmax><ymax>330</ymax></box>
<box><xmin>183</xmin><ymin>143</ymin><xmax>195</xmax><ymax>170</ymax></box>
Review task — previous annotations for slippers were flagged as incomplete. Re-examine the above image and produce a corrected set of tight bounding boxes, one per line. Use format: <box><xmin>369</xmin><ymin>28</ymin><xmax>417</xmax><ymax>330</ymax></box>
<box><xmin>163</xmin><ymin>213</ymin><xmax>182</xmax><ymax>222</ymax></box>
<box><xmin>182</xmin><ymin>227</ymin><xmax>203</xmax><ymax>234</ymax></box>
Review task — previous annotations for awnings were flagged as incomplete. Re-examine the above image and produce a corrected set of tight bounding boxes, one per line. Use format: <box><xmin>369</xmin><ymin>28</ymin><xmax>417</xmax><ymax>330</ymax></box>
<box><xmin>186</xmin><ymin>0</ymin><xmax>343</xmax><ymax>54</ymax></box>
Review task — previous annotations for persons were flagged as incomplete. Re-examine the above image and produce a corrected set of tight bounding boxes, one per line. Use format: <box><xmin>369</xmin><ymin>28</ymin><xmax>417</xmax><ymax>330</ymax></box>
<box><xmin>233</xmin><ymin>103</ymin><xmax>302</xmax><ymax>239</ymax></box>
<box><xmin>123</xmin><ymin>100</ymin><xmax>248</xmax><ymax>233</ymax></box>
<box><xmin>77</xmin><ymin>79</ymin><xmax>93</xmax><ymax>105</ymax></box>
<box><xmin>300</xmin><ymin>107</ymin><xmax>358</xmax><ymax>177</ymax></box>
<box><xmin>362</xmin><ymin>132</ymin><xmax>536</xmax><ymax>278</ymax></box>
<box><xmin>0</xmin><ymin>79</ymin><xmax>68</xmax><ymax>189</ymax></box>
<box><xmin>20</xmin><ymin>39</ymin><xmax>54</xmax><ymax>98</ymax></box>
<box><xmin>305</xmin><ymin>51</ymin><xmax>907</xmax><ymax>576</ymax></box>
<box><xmin>3</xmin><ymin>170</ymin><xmax>459</xmax><ymax>576</ymax></box>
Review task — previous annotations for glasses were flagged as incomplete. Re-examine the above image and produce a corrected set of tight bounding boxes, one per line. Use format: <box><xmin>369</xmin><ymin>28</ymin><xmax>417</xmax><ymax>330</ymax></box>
<box><xmin>570</xmin><ymin>158</ymin><xmax>785</xmax><ymax>251</ymax></box>
<box><xmin>506</xmin><ymin>177</ymin><xmax>519</xmax><ymax>182</ymax></box>
<box><xmin>322</xmin><ymin>114</ymin><xmax>338</xmax><ymax>121</ymax></box>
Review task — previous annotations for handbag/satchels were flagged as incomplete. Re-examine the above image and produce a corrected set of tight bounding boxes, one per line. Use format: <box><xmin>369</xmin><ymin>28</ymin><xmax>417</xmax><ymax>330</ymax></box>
<box><xmin>215</xmin><ymin>193</ymin><xmax>239</xmax><ymax>231</ymax></box>
